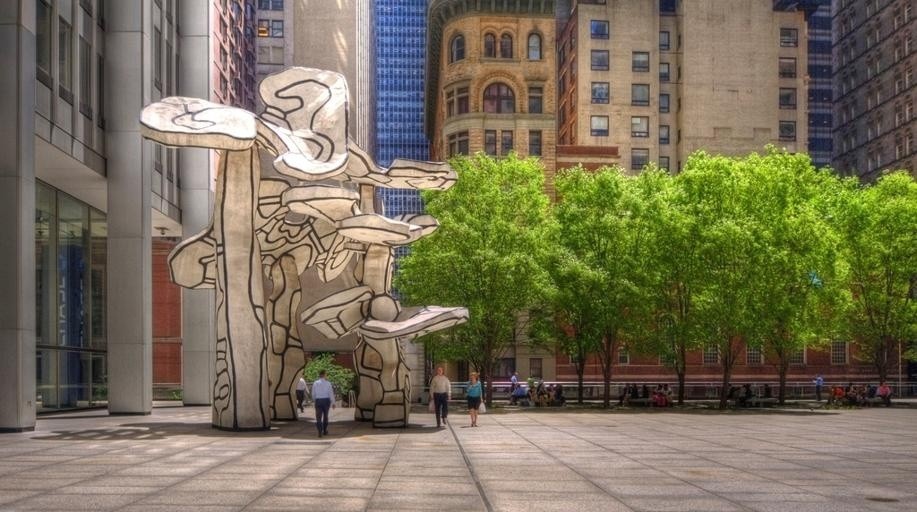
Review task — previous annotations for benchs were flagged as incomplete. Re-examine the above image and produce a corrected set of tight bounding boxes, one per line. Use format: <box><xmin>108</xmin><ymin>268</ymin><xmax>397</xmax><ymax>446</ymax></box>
<box><xmin>628</xmin><ymin>398</ymin><xmax>654</xmax><ymax>406</ymax></box>
<box><xmin>862</xmin><ymin>397</ymin><xmax>882</xmax><ymax>406</ymax></box>
<box><xmin>727</xmin><ymin>397</ymin><xmax>776</xmax><ymax>409</ymax></box>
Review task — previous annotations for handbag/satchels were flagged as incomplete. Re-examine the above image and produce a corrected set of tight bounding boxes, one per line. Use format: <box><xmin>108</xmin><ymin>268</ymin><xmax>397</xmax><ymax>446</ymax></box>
<box><xmin>428</xmin><ymin>399</ymin><xmax>436</xmax><ymax>411</ymax></box>
<box><xmin>478</xmin><ymin>402</ymin><xmax>486</xmax><ymax>413</ymax></box>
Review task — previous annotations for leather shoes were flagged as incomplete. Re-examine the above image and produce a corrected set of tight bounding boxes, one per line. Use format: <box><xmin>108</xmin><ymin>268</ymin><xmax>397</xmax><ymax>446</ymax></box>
<box><xmin>318</xmin><ymin>430</ymin><xmax>328</xmax><ymax>437</ymax></box>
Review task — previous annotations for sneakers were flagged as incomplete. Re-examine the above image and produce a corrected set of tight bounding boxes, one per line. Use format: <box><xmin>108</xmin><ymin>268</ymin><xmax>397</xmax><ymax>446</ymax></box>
<box><xmin>436</xmin><ymin>417</ymin><xmax>447</xmax><ymax>427</ymax></box>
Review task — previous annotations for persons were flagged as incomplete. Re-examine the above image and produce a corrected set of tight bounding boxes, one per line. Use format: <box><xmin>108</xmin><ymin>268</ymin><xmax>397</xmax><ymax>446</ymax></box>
<box><xmin>467</xmin><ymin>373</ymin><xmax>483</xmax><ymax>427</ymax></box>
<box><xmin>812</xmin><ymin>372</ymin><xmax>823</xmax><ymax>401</ymax></box>
<box><xmin>312</xmin><ymin>370</ymin><xmax>336</xmax><ymax>437</ymax></box>
<box><xmin>430</xmin><ymin>367</ymin><xmax>451</xmax><ymax>428</ymax></box>
<box><xmin>619</xmin><ymin>383</ymin><xmax>673</xmax><ymax>407</ymax></box>
<box><xmin>509</xmin><ymin>371</ymin><xmax>566</xmax><ymax>407</ymax></box>
<box><xmin>728</xmin><ymin>384</ymin><xmax>771</xmax><ymax>408</ymax></box>
<box><xmin>826</xmin><ymin>379</ymin><xmax>891</xmax><ymax>407</ymax></box>
<box><xmin>295</xmin><ymin>374</ymin><xmax>310</xmax><ymax>413</ymax></box>
